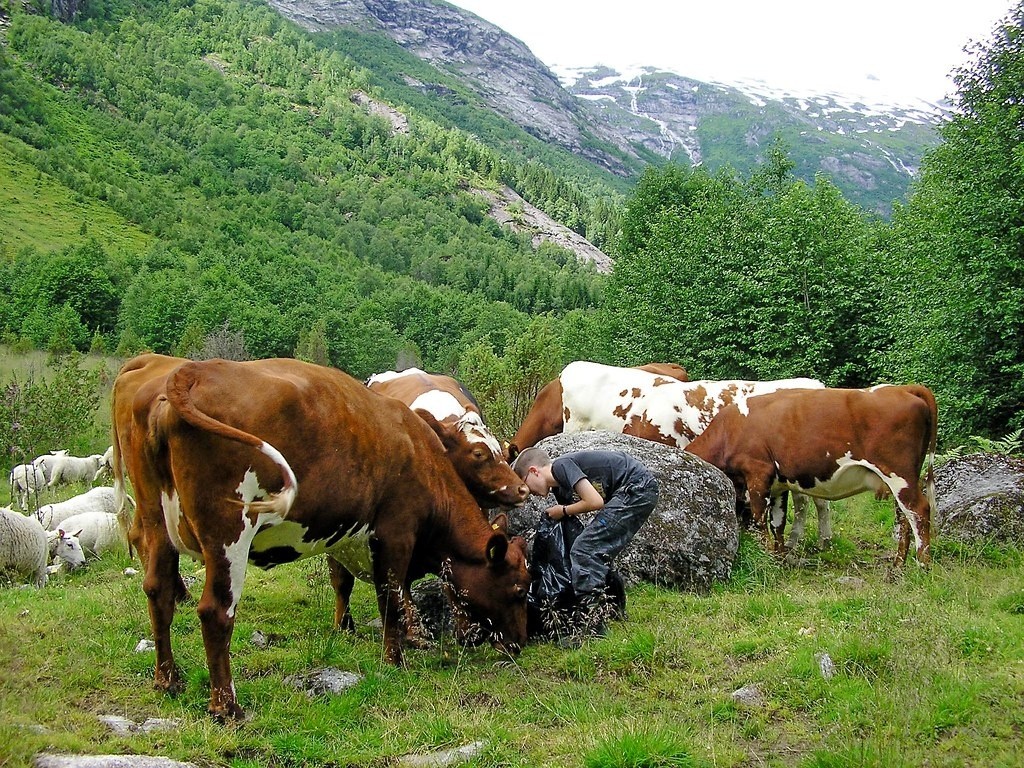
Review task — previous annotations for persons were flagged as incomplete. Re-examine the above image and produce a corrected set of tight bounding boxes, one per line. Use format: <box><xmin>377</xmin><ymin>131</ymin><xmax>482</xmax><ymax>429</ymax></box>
<box><xmin>514</xmin><ymin>446</ymin><xmax>658</xmax><ymax>630</ymax></box>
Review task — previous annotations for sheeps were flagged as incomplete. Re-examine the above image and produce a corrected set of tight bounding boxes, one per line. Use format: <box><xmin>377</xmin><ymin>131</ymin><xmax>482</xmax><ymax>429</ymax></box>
<box><xmin>0</xmin><ymin>445</ymin><xmax>136</xmax><ymax>590</ymax></box>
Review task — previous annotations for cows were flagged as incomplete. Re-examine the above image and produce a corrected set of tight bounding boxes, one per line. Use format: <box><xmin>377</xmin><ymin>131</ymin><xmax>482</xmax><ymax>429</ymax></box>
<box><xmin>111</xmin><ymin>342</ymin><xmax>943</xmax><ymax>725</ymax></box>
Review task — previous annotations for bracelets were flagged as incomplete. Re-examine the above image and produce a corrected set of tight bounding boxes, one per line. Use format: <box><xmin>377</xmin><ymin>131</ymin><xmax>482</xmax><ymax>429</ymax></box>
<box><xmin>562</xmin><ymin>504</ymin><xmax>570</xmax><ymax>517</ymax></box>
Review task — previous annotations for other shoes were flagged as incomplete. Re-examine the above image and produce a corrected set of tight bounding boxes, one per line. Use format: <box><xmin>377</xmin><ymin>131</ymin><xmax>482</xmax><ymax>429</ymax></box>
<box><xmin>557</xmin><ymin>628</ymin><xmax>612</xmax><ymax>649</ymax></box>
